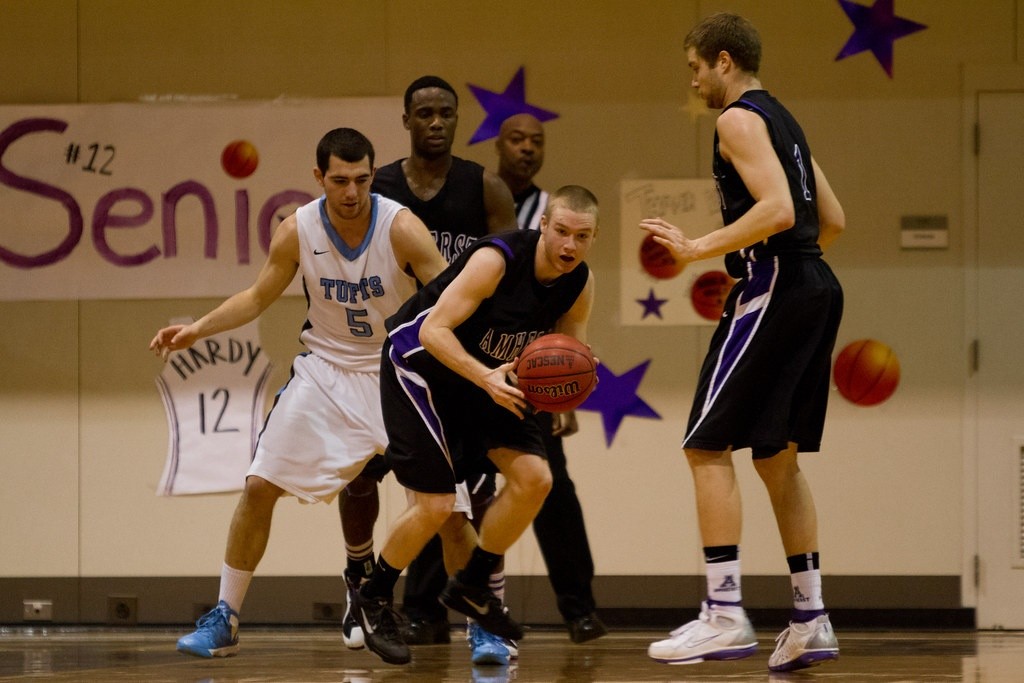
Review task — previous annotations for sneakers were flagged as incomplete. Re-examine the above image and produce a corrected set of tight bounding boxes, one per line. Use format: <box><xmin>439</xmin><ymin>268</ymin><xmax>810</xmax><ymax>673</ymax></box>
<box><xmin>768</xmin><ymin>613</ymin><xmax>841</xmax><ymax>672</ymax></box>
<box><xmin>176</xmin><ymin>600</ymin><xmax>239</xmax><ymax>658</ymax></box>
<box><xmin>500</xmin><ymin>635</ymin><xmax>518</xmax><ymax>658</ymax></box>
<box><xmin>341</xmin><ymin>568</ymin><xmax>370</xmax><ymax>651</ymax></box>
<box><xmin>467</xmin><ymin>619</ymin><xmax>510</xmax><ymax>665</ymax></box>
<box><xmin>349</xmin><ymin>580</ymin><xmax>411</xmax><ymax>665</ymax></box>
<box><xmin>648</xmin><ymin>600</ymin><xmax>758</xmax><ymax>666</ymax></box>
<box><xmin>441</xmin><ymin>568</ymin><xmax>524</xmax><ymax>641</ymax></box>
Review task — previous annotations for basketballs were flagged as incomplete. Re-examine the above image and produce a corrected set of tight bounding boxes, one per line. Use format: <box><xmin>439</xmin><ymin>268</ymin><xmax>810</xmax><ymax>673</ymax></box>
<box><xmin>640</xmin><ymin>232</ymin><xmax>685</xmax><ymax>281</ymax></box>
<box><xmin>221</xmin><ymin>139</ymin><xmax>259</xmax><ymax>178</ymax></box>
<box><xmin>833</xmin><ymin>338</ymin><xmax>903</xmax><ymax>408</ymax></box>
<box><xmin>515</xmin><ymin>333</ymin><xmax>599</xmax><ymax>414</ymax></box>
<box><xmin>691</xmin><ymin>270</ymin><xmax>735</xmax><ymax>323</ymax></box>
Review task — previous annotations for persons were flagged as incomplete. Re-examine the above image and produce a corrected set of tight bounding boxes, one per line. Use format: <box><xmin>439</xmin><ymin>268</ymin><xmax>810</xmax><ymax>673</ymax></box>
<box><xmin>150</xmin><ymin>125</ymin><xmax>515</xmax><ymax>666</ymax></box>
<box><xmin>388</xmin><ymin>111</ymin><xmax>605</xmax><ymax>639</ymax></box>
<box><xmin>638</xmin><ymin>10</ymin><xmax>847</xmax><ymax>669</ymax></box>
<box><xmin>354</xmin><ymin>183</ymin><xmax>600</xmax><ymax>665</ymax></box>
<box><xmin>334</xmin><ymin>73</ymin><xmax>520</xmax><ymax>658</ymax></box>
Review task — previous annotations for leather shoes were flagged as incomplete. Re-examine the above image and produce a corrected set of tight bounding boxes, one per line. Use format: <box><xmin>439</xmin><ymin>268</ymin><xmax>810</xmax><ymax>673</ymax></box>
<box><xmin>566</xmin><ymin>610</ymin><xmax>606</xmax><ymax>644</ymax></box>
<box><xmin>391</xmin><ymin>601</ymin><xmax>451</xmax><ymax>646</ymax></box>
